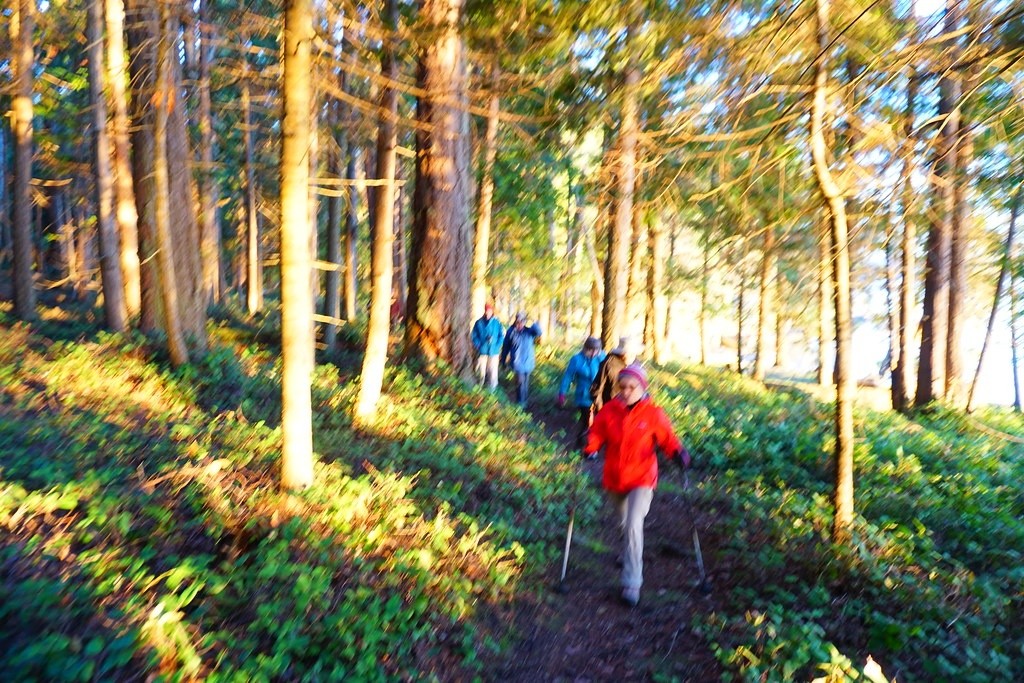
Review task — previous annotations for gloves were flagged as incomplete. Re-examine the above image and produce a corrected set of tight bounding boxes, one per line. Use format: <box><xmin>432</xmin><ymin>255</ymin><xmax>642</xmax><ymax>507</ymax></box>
<box><xmin>576</xmin><ymin>427</ymin><xmax>591</xmax><ymax>448</ymax></box>
<box><xmin>678</xmin><ymin>448</ymin><xmax>691</xmax><ymax>471</ymax></box>
<box><xmin>559</xmin><ymin>395</ymin><xmax>565</xmax><ymax>404</ymax></box>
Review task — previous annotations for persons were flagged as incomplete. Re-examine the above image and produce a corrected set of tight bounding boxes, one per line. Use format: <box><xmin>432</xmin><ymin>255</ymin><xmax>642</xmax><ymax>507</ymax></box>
<box><xmin>499</xmin><ymin>310</ymin><xmax>542</xmax><ymax>412</ymax></box>
<box><xmin>589</xmin><ymin>348</ymin><xmax>627</xmax><ymax>416</ymax></box>
<box><xmin>470</xmin><ymin>303</ymin><xmax>506</xmax><ymax>389</ymax></box>
<box><xmin>558</xmin><ymin>337</ymin><xmax>607</xmax><ymax>459</ymax></box>
<box><xmin>581</xmin><ymin>363</ymin><xmax>692</xmax><ymax>607</ymax></box>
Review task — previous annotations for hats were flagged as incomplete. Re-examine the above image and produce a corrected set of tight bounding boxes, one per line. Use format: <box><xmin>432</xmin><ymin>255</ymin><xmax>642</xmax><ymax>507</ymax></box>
<box><xmin>485</xmin><ymin>303</ymin><xmax>493</xmax><ymax>313</ymax></box>
<box><xmin>584</xmin><ymin>337</ymin><xmax>602</xmax><ymax>349</ymax></box>
<box><xmin>516</xmin><ymin>311</ymin><xmax>526</xmax><ymax>321</ymax></box>
<box><xmin>612</xmin><ymin>336</ymin><xmax>646</xmax><ymax>355</ymax></box>
<box><xmin>618</xmin><ymin>360</ymin><xmax>649</xmax><ymax>389</ymax></box>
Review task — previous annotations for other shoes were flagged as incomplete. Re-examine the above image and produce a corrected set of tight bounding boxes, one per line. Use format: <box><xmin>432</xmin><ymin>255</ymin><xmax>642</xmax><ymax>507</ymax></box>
<box><xmin>614</xmin><ymin>553</ymin><xmax>623</xmax><ymax>568</ymax></box>
<box><xmin>620</xmin><ymin>585</ymin><xmax>640</xmax><ymax>606</ymax></box>
